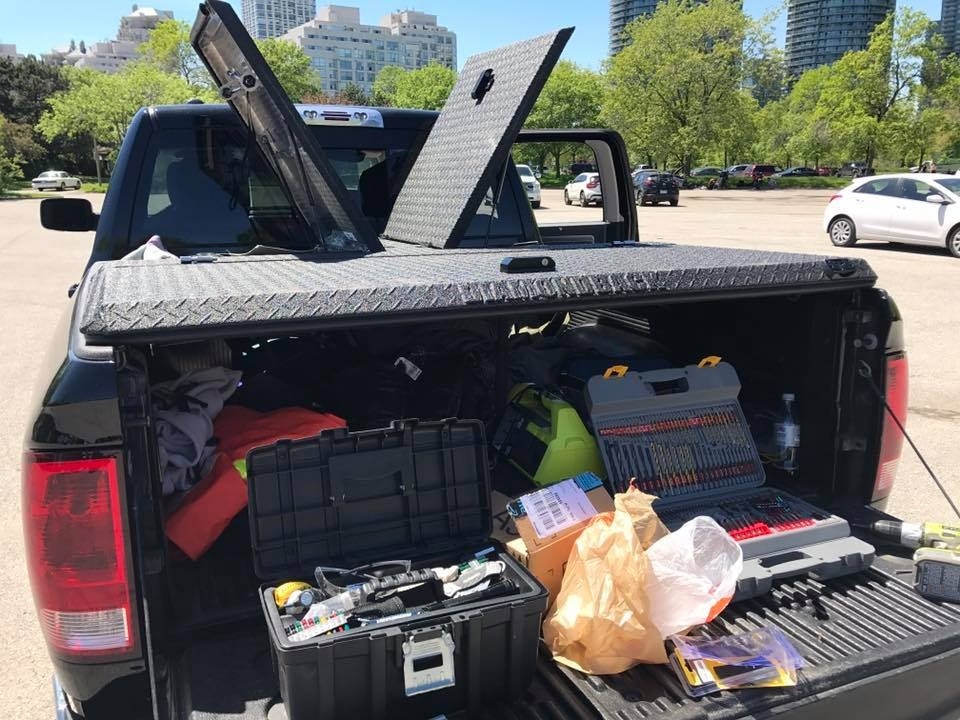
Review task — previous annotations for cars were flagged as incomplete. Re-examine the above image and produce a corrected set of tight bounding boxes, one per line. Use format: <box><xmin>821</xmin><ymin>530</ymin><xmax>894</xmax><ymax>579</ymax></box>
<box><xmin>533</xmin><ymin>165</ymin><xmax>549</xmax><ymax>174</ymax></box>
<box><xmin>718</xmin><ymin>163</ymin><xmax>784</xmax><ymax>179</ymax></box>
<box><xmin>630</xmin><ymin>164</ymin><xmax>685</xmax><ymax>206</ymax></box>
<box><xmin>689</xmin><ymin>166</ymin><xmax>723</xmax><ymax>177</ymax></box>
<box><xmin>823</xmin><ymin>172</ymin><xmax>960</xmax><ymax>257</ymax></box>
<box><xmin>564</xmin><ymin>172</ymin><xmax>602</xmax><ymax>207</ymax></box>
<box><xmin>31</xmin><ymin>170</ymin><xmax>82</xmax><ymax>191</ymax></box>
<box><xmin>831</xmin><ymin>161</ymin><xmax>874</xmax><ymax>177</ymax></box>
<box><xmin>483</xmin><ymin>164</ymin><xmax>541</xmax><ymax>209</ymax></box>
<box><xmin>771</xmin><ymin>166</ymin><xmax>834</xmax><ymax>177</ymax></box>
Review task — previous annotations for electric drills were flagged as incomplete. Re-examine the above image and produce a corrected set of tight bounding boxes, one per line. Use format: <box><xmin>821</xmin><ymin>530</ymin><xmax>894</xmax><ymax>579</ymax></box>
<box><xmin>852</xmin><ymin>519</ymin><xmax>960</xmax><ymax>603</ymax></box>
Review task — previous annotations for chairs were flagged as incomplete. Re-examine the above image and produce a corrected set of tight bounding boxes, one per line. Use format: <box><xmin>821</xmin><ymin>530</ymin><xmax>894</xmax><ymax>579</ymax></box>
<box><xmin>148</xmin><ymin>157</ymin><xmax>260</xmax><ymax>251</ymax></box>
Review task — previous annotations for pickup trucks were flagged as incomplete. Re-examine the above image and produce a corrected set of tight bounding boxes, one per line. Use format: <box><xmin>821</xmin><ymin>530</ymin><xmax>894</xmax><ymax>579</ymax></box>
<box><xmin>21</xmin><ymin>0</ymin><xmax>960</xmax><ymax>720</ymax></box>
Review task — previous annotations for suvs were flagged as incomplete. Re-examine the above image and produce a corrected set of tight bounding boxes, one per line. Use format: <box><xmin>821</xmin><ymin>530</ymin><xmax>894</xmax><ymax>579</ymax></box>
<box><xmin>567</xmin><ymin>163</ymin><xmax>599</xmax><ymax>174</ymax></box>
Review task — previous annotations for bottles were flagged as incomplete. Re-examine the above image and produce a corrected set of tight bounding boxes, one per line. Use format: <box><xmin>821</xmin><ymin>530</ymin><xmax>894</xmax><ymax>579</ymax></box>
<box><xmin>771</xmin><ymin>394</ymin><xmax>800</xmax><ymax>484</ymax></box>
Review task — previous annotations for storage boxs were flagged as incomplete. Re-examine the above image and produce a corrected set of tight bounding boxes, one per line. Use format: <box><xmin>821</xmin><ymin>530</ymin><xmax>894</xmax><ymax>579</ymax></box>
<box><xmin>488</xmin><ymin>469</ymin><xmax>615</xmax><ymax>613</ymax></box>
<box><xmin>245</xmin><ymin>417</ymin><xmax>550</xmax><ymax>720</ymax></box>
<box><xmin>585</xmin><ymin>357</ymin><xmax>875</xmax><ymax>604</ymax></box>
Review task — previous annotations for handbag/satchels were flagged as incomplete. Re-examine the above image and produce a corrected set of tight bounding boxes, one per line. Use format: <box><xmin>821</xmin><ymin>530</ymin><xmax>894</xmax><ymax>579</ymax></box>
<box><xmin>538</xmin><ymin>477</ymin><xmax>667</xmax><ymax>674</ymax></box>
<box><xmin>642</xmin><ymin>516</ymin><xmax>744</xmax><ymax>642</ymax></box>
